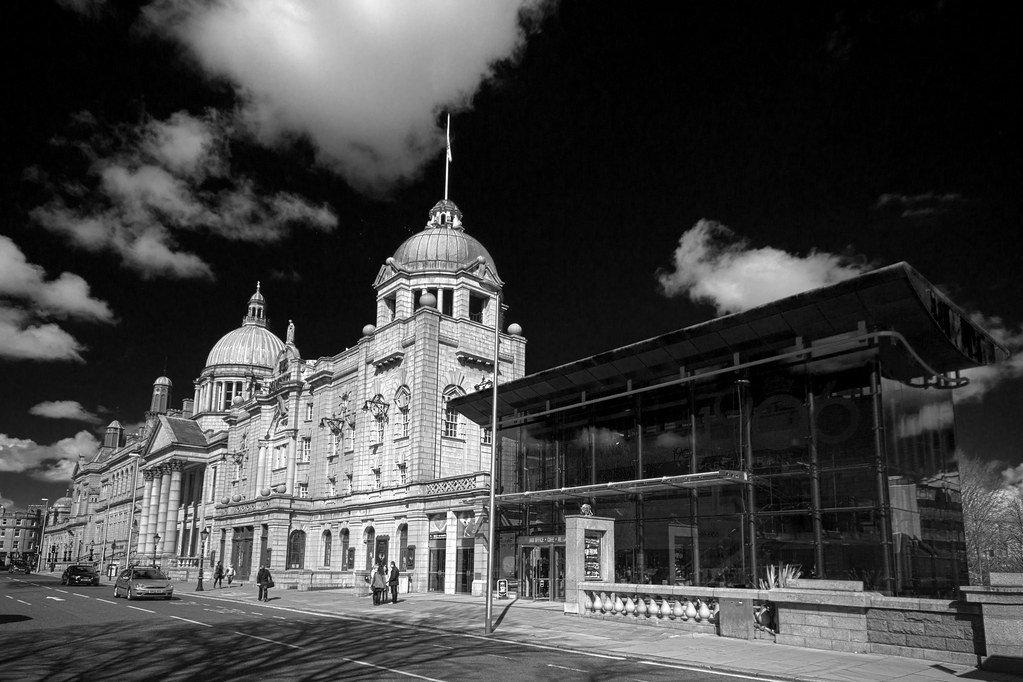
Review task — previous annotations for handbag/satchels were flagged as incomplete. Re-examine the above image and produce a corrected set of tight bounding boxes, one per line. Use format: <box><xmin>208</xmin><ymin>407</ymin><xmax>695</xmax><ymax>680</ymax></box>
<box><xmin>214</xmin><ymin>573</ymin><xmax>219</xmax><ymax>579</ymax></box>
<box><xmin>267</xmin><ymin>579</ymin><xmax>274</xmax><ymax>588</ymax></box>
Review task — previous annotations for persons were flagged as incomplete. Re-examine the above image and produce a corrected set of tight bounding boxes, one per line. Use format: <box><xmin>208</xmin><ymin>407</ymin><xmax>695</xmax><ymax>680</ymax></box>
<box><xmin>224</xmin><ymin>564</ymin><xmax>236</xmax><ymax>589</ymax></box>
<box><xmin>388</xmin><ymin>562</ymin><xmax>400</xmax><ymax>606</ymax></box>
<box><xmin>381</xmin><ymin>564</ymin><xmax>390</xmax><ymax>604</ymax></box>
<box><xmin>257</xmin><ymin>565</ymin><xmax>272</xmax><ymax>603</ymax></box>
<box><xmin>213</xmin><ymin>562</ymin><xmax>225</xmax><ymax>590</ymax></box>
<box><xmin>370</xmin><ymin>566</ymin><xmax>386</xmax><ymax>607</ymax></box>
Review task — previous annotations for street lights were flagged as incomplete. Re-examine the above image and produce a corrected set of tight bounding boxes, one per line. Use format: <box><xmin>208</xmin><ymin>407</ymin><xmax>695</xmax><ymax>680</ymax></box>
<box><xmin>478</xmin><ymin>279</ymin><xmax>500</xmax><ymax>635</ymax></box>
<box><xmin>36</xmin><ymin>498</ymin><xmax>49</xmax><ymax>573</ymax></box>
<box><xmin>108</xmin><ymin>539</ymin><xmax>116</xmax><ymax>581</ymax></box>
<box><xmin>126</xmin><ymin>453</ymin><xmax>141</xmax><ymax>573</ymax></box>
<box><xmin>151</xmin><ymin>533</ymin><xmax>161</xmax><ymax>568</ymax></box>
<box><xmin>47</xmin><ymin>539</ymin><xmax>95</xmax><ymax>563</ymax></box>
<box><xmin>195</xmin><ymin>526</ymin><xmax>212</xmax><ymax>591</ymax></box>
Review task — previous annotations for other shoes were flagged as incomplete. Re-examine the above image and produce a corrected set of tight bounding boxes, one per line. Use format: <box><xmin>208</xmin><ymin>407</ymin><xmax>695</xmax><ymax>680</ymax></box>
<box><xmin>388</xmin><ymin>602</ymin><xmax>394</xmax><ymax>604</ymax></box>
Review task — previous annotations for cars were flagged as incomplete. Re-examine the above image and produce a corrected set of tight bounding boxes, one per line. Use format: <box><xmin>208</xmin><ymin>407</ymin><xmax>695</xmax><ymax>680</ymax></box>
<box><xmin>62</xmin><ymin>565</ymin><xmax>100</xmax><ymax>586</ymax></box>
<box><xmin>113</xmin><ymin>564</ymin><xmax>173</xmax><ymax>601</ymax></box>
<box><xmin>9</xmin><ymin>560</ymin><xmax>30</xmax><ymax>575</ymax></box>
